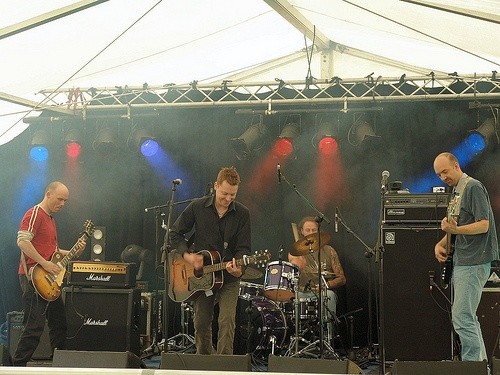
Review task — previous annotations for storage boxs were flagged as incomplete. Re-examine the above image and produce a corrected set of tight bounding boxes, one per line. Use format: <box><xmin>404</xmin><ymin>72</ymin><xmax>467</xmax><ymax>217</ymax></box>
<box><xmin>62</xmin><ymin>287</ymin><xmax>154</xmax><ymax>359</ymax></box>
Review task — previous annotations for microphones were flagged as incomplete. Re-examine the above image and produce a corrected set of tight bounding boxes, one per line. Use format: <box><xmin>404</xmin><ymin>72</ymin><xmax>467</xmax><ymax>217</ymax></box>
<box><xmin>381</xmin><ymin>170</ymin><xmax>390</xmax><ymax>189</ymax></box>
<box><xmin>277</xmin><ymin>163</ymin><xmax>281</xmax><ymax>183</ymax></box>
<box><xmin>172</xmin><ymin>179</ymin><xmax>182</xmax><ymax>185</ymax></box>
<box><xmin>334</xmin><ymin>208</ymin><xmax>338</xmax><ymax>232</ymax></box>
<box><xmin>304</xmin><ymin>278</ymin><xmax>311</xmax><ymax>291</ymax></box>
<box><xmin>210</xmin><ymin>183</ymin><xmax>213</xmax><ymax>197</ymax></box>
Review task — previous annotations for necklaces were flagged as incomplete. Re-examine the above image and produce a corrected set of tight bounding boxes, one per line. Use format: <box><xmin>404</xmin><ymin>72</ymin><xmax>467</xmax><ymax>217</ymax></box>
<box><xmin>215</xmin><ymin>204</ymin><xmax>227</xmax><ymax>219</ymax></box>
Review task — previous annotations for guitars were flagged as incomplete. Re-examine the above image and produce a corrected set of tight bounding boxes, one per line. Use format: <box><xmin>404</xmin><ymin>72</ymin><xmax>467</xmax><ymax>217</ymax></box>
<box><xmin>442</xmin><ymin>192</ymin><xmax>460</xmax><ymax>283</ymax></box>
<box><xmin>28</xmin><ymin>219</ymin><xmax>96</xmax><ymax>301</ymax></box>
<box><xmin>167</xmin><ymin>247</ymin><xmax>273</xmax><ymax>302</ymax></box>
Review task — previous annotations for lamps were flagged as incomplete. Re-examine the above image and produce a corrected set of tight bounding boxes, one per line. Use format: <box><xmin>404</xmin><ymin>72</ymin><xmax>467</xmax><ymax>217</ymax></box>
<box><xmin>28</xmin><ymin>112</ymin><xmax>388</xmax><ymax>166</ymax></box>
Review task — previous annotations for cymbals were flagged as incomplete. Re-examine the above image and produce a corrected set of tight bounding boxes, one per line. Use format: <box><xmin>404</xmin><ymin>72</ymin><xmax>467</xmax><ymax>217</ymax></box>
<box><xmin>239</xmin><ymin>266</ymin><xmax>264</xmax><ymax>280</ymax></box>
<box><xmin>306</xmin><ymin>270</ymin><xmax>344</xmax><ymax>280</ymax></box>
<box><xmin>290</xmin><ymin>232</ymin><xmax>330</xmax><ymax>256</ymax></box>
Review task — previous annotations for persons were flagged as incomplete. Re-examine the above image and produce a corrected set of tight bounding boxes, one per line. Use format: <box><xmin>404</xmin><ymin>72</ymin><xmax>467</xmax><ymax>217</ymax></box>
<box><xmin>169</xmin><ymin>168</ymin><xmax>251</xmax><ymax>355</ymax></box>
<box><xmin>288</xmin><ymin>217</ymin><xmax>346</xmax><ymax>348</ymax></box>
<box><xmin>433</xmin><ymin>152</ymin><xmax>500</xmax><ymax>375</ymax></box>
<box><xmin>13</xmin><ymin>181</ymin><xmax>86</xmax><ymax>366</ymax></box>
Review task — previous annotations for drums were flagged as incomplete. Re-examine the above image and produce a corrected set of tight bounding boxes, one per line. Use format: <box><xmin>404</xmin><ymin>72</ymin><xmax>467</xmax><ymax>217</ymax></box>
<box><xmin>239</xmin><ymin>280</ymin><xmax>264</xmax><ymax>298</ymax></box>
<box><xmin>262</xmin><ymin>259</ymin><xmax>300</xmax><ymax>302</ymax></box>
<box><xmin>212</xmin><ymin>296</ymin><xmax>288</xmax><ymax>358</ymax></box>
<box><xmin>281</xmin><ymin>297</ymin><xmax>318</xmax><ymax>320</ymax></box>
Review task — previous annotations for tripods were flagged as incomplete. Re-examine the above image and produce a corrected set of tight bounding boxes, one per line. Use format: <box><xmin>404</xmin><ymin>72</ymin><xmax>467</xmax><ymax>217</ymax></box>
<box><xmin>158</xmin><ymin>303</ymin><xmax>194</xmax><ymax>354</ymax></box>
<box><xmin>281</xmin><ymin>172</ymin><xmax>343</xmax><ymax>362</ymax></box>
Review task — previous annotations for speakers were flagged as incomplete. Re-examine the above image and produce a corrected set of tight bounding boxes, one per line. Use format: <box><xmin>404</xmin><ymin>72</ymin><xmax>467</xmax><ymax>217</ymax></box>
<box><xmin>6</xmin><ymin>287</ymin><xmax>148</xmax><ymax>370</ymax></box>
<box><xmin>267</xmin><ymin>354</ymin><xmax>365</xmax><ymax>375</ymax></box>
<box><xmin>160</xmin><ymin>351</ymin><xmax>251</xmax><ymax>373</ymax></box>
<box><xmin>380</xmin><ymin>224</ymin><xmax>500</xmax><ymax>375</ymax></box>
